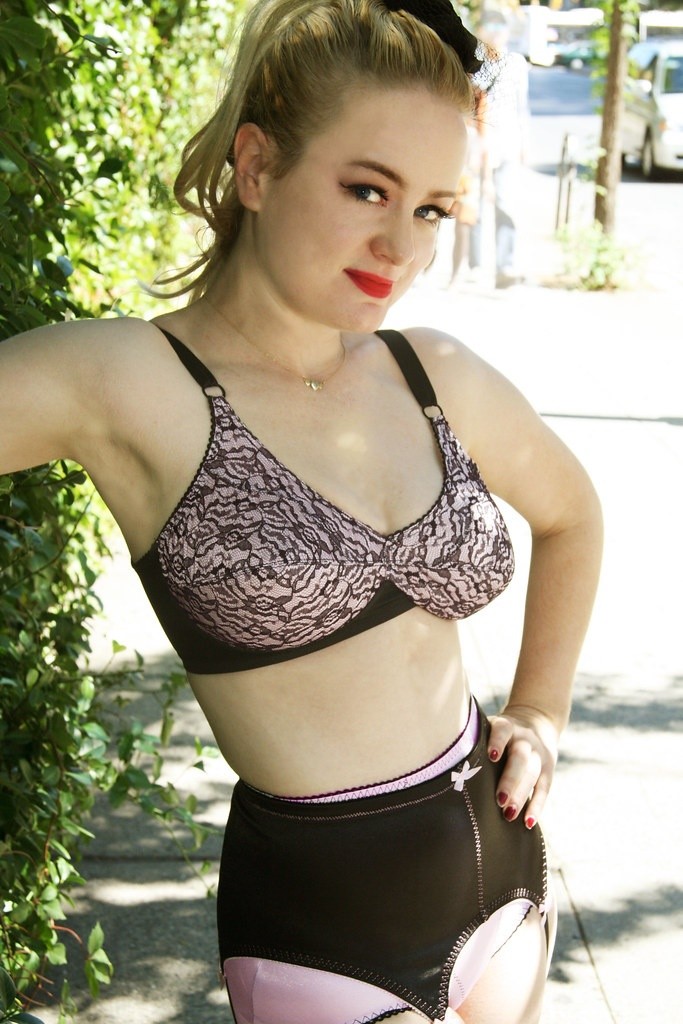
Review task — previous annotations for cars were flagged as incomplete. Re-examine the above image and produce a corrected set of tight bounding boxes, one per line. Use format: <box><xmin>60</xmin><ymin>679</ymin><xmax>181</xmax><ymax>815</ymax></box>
<box><xmin>525</xmin><ymin>25</ymin><xmax>683</xmax><ymax>176</ymax></box>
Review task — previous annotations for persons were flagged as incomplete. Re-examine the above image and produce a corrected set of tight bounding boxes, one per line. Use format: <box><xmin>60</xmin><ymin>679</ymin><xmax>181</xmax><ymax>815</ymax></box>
<box><xmin>0</xmin><ymin>0</ymin><xmax>607</xmax><ymax>1024</ymax></box>
